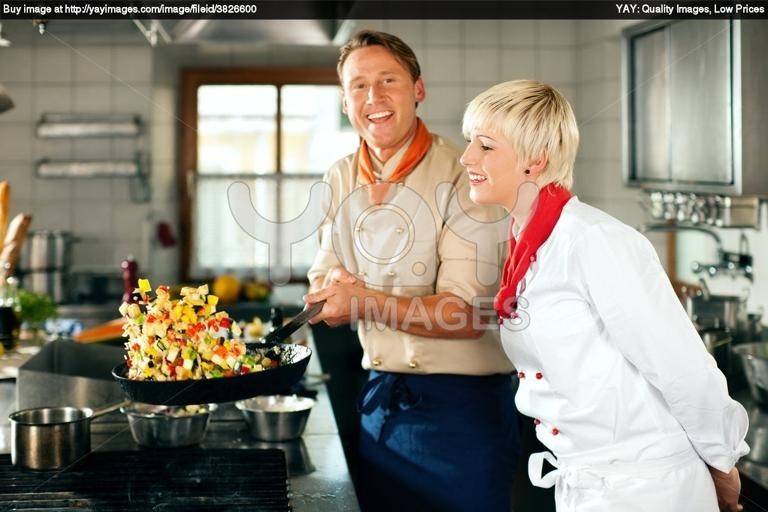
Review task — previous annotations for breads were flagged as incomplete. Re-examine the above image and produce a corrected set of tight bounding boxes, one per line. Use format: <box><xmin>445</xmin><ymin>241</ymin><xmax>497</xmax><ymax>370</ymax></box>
<box><xmin>0</xmin><ymin>180</ymin><xmax>32</xmax><ymax>273</ymax></box>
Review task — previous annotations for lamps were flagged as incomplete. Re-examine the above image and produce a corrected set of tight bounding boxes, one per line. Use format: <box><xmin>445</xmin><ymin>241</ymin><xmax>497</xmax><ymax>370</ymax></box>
<box><xmin>0</xmin><ymin>22</ymin><xmax>15</xmax><ymax>49</ymax></box>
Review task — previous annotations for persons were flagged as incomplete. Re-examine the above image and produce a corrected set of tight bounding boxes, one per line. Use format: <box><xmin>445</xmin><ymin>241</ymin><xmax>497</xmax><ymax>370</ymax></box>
<box><xmin>459</xmin><ymin>79</ymin><xmax>751</xmax><ymax>512</ymax></box>
<box><xmin>303</xmin><ymin>30</ymin><xmax>519</xmax><ymax>512</ymax></box>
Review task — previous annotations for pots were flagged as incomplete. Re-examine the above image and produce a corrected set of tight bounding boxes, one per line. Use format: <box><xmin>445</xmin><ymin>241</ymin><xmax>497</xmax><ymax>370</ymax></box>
<box><xmin>19</xmin><ymin>270</ymin><xmax>72</xmax><ymax>309</ymax></box>
<box><xmin>8</xmin><ymin>400</ymin><xmax>131</xmax><ymax>470</ymax></box>
<box><xmin>679</xmin><ymin>279</ymin><xmax>752</xmax><ymax>332</ymax></box>
<box><xmin>111</xmin><ymin>300</ymin><xmax>331</xmax><ymax>405</ymax></box>
<box><xmin>17</xmin><ymin>231</ymin><xmax>76</xmax><ymax>271</ymax></box>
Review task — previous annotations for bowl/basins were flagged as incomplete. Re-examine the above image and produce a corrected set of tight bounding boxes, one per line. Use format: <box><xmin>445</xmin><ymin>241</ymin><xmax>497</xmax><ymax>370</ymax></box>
<box><xmin>733</xmin><ymin>340</ymin><xmax>767</xmax><ymax>408</ymax></box>
<box><xmin>121</xmin><ymin>406</ymin><xmax>218</xmax><ymax>450</ymax></box>
<box><xmin>741</xmin><ymin>412</ymin><xmax>768</xmax><ymax>468</ymax></box>
<box><xmin>241</xmin><ymin>441</ymin><xmax>314</xmax><ymax>477</ymax></box>
<box><xmin>235</xmin><ymin>396</ymin><xmax>318</xmax><ymax>442</ymax></box>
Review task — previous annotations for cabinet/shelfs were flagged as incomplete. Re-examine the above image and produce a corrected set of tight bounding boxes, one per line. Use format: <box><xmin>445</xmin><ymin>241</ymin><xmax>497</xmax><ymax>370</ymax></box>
<box><xmin>620</xmin><ymin>20</ymin><xmax>768</xmax><ymax>200</ymax></box>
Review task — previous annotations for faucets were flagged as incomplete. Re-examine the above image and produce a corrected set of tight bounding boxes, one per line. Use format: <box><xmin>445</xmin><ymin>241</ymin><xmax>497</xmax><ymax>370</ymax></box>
<box><xmin>636</xmin><ymin>220</ymin><xmax>724</xmax><ymax>260</ymax></box>
